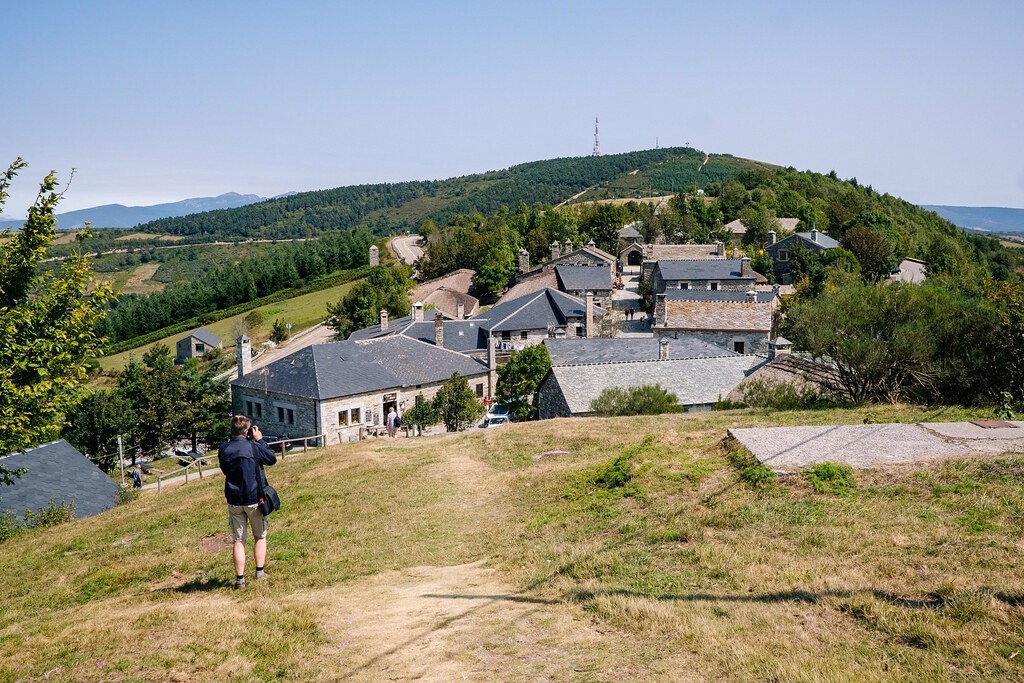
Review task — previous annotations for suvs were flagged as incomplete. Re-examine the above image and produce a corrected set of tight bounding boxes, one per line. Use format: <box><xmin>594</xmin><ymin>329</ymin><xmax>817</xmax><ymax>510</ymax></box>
<box><xmin>484</xmin><ymin>403</ymin><xmax>514</xmax><ymax>426</ymax></box>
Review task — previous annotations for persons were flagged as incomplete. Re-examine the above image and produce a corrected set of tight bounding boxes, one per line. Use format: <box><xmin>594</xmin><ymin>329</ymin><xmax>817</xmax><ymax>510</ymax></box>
<box><xmin>131</xmin><ymin>464</ymin><xmax>142</xmax><ymax>487</ymax></box>
<box><xmin>386</xmin><ymin>407</ymin><xmax>398</xmax><ymax>438</ymax></box>
<box><xmin>218</xmin><ymin>415</ymin><xmax>277</xmax><ymax>591</ymax></box>
<box><xmin>483</xmin><ymin>396</ymin><xmax>492</xmax><ymax>411</ymax></box>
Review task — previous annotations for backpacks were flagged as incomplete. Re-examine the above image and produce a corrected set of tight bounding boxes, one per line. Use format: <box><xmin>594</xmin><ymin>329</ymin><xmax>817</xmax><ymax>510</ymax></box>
<box><xmin>394</xmin><ymin>416</ymin><xmax>400</xmax><ymax>428</ymax></box>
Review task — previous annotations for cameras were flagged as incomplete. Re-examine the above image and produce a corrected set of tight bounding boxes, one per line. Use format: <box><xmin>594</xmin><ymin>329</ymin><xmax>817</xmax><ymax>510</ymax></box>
<box><xmin>246</xmin><ymin>425</ymin><xmax>255</xmax><ymax>440</ymax></box>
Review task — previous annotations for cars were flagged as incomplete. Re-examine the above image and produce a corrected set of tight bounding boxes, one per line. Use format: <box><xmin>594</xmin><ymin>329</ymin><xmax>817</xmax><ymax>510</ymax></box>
<box><xmin>263</xmin><ymin>436</ymin><xmax>294</xmax><ymax>452</ymax></box>
<box><xmin>486</xmin><ymin>416</ymin><xmax>510</xmax><ymax>429</ymax></box>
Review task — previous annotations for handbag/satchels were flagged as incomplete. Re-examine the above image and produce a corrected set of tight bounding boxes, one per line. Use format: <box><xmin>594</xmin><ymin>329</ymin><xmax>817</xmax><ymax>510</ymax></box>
<box><xmin>259</xmin><ymin>485</ymin><xmax>280</xmax><ymax>517</ymax></box>
<box><xmin>135</xmin><ymin>478</ymin><xmax>141</xmax><ymax>486</ymax></box>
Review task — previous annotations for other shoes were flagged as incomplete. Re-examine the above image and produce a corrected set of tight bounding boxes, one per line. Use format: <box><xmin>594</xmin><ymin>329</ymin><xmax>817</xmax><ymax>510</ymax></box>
<box><xmin>256</xmin><ymin>573</ymin><xmax>270</xmax><ymax>579</ymax></box>
<box><xmin>235</xmin><ymin>583</ymin><xmax>246</xmax><ymax>589</ymax></box>
